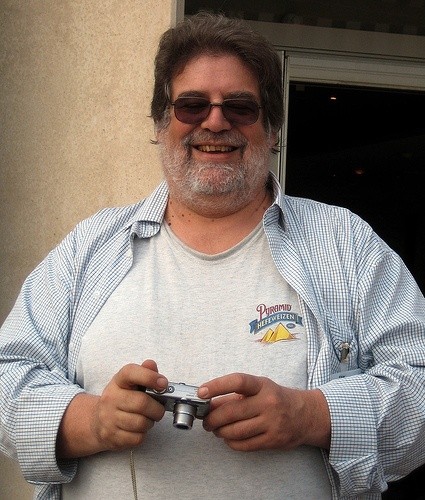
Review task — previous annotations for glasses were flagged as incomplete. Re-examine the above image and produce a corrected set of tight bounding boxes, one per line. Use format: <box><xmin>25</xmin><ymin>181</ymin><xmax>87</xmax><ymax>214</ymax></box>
<box><xmin>168</xmin><ymin>96</ymin><xmax>267</xmax><ymax>125</ymax></box>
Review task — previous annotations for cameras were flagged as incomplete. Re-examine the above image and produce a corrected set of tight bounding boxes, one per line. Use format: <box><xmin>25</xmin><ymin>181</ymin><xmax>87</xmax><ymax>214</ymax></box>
<box><xmin>145</xmin><ymin>380</ymin><xmax>211</xmax><ymax>431</ymax></box>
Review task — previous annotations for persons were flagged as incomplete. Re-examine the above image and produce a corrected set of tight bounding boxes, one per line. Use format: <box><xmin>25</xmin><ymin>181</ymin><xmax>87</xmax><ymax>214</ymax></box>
<box><xmin>0</xmin><ymin>14</ymin><xmax>425</xmax><ymax>499</ymax></box>
